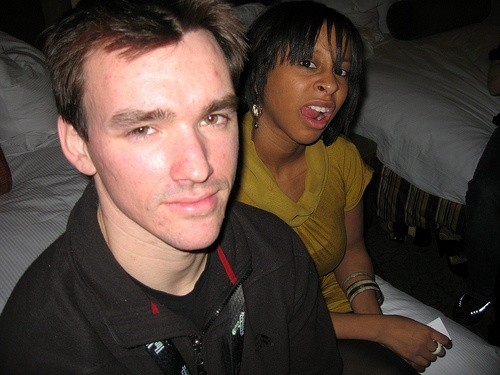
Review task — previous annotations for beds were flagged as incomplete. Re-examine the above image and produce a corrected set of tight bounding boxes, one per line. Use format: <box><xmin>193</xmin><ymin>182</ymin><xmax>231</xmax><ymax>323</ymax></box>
<box><xmin>0</xmin><ymin>0</ymin><xmax>500</xmax><ymax>375</ymax></box>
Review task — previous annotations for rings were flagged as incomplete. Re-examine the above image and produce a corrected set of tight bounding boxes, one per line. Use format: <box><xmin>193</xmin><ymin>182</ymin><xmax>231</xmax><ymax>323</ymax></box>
<box><xmin>432</xmin><ymin>342</ymin><xmax>441</xmax><ymax>354</ymax></box>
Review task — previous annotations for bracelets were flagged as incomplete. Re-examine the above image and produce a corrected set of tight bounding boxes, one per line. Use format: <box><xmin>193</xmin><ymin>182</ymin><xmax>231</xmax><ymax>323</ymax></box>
<box><xmin>342</xmin><ymin>272</ymin><xmax>385</xmax><ymax>307</ymax></box>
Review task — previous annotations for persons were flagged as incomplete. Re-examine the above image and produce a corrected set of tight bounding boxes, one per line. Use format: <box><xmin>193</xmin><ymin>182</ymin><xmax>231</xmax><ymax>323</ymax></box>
<box><xmin>229</xmin><ymin>0</ymin><xmax>454</xmax><ymax>375</ymax></box>
<box><xmin>0</xmin><ymin>0</ymin><xmax>345</xmax><ymax>375</ymax></box>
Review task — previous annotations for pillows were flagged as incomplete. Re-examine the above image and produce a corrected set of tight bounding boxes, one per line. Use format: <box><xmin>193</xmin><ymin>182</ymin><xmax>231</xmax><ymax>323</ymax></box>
<box><xmin>323</xmin><ymin>0</ymin><xmax>397</xmax><ymax>40</ymax></box>
<box><xmin>0</xmin><ymin>32</ymin><xmax>60</xmax><ymax>143</ymax></box>
